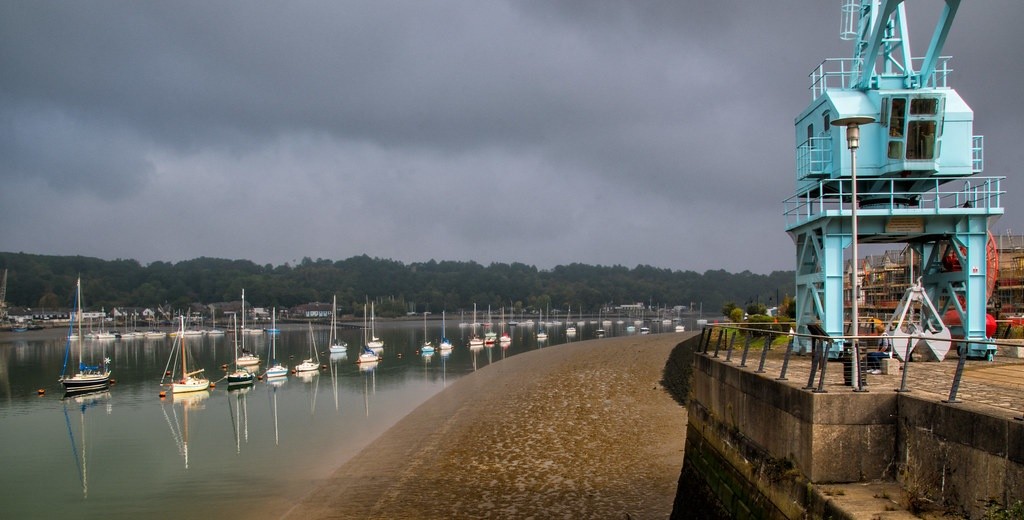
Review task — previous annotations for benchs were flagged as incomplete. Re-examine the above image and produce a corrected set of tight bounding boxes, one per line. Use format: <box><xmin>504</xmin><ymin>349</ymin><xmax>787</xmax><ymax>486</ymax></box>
<box><xmin>881</xmin><ymin>358</ymin><xmax>899</xmax><ymax>375</ymax></box>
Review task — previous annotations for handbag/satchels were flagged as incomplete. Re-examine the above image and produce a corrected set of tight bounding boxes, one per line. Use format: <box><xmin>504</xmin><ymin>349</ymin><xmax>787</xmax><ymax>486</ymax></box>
<box><xmin>879</xmin><ymin>334</ymin><xmax>891</xmax><ymax>352</ymax></box>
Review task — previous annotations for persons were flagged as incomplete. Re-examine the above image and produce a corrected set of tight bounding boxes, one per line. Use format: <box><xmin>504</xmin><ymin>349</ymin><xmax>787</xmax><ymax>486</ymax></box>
<box><xmin>868</xmin><ymin>325</ymin><xmax>893</xmax><ymax>374</ymax></box>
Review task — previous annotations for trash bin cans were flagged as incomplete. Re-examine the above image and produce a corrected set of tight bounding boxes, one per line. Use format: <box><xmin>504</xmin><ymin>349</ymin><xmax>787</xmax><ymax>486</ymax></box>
<box><xmin>843</xmin><ymin>341</ymin><xmax>868</xmax><ymax>386</ymax></box>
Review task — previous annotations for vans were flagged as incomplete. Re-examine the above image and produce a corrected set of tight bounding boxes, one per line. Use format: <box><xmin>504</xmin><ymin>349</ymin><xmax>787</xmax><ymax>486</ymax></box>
<box><xmin>105</xmin><ymin>318</ymin><xmax>112</xmax><ymax>321</ymax></box>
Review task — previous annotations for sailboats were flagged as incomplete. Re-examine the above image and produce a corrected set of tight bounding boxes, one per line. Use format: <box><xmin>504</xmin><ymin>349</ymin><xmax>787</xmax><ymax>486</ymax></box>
<box><xmin>640</xmin><ymin>309</ymin><xmax>649</xmax><ymax>330</ymax></box>
<box><xmin>358</xmin><ymin>304</ymin><xmax>379</xmax><ymax>363</ymax></box>
<box><xmin>227</xmin><ymin>382</ymin><xmax>251</xmax><ymax>454</ymax></box>
<box><xmin>58</xmin><ymin>274</ymin><xmax>114</xmax><ymax>395</ymax></box>
<box><xmin>625</xmin><ymin>307</ymin><xmax>635</xmax><ymax>329</ymax></box>
<box><xmin>66</xmin><ymin>306</ymin><xmax>280</xmax><ymax>340</ymax></box>
<box><xmin>500</xmin><ymin>306</ymin><xmax>511</xmax><ymax>342</ymax></box>
<box><xmin>596</xmin><ymin>308</ymin><xmax>605</xmax><ymax>334</ymax></box>
<box><xmin>468</xmin><ymin>345</ymin><xmax>483</xmax><ymax>372</ymax></box>
<box><xmin>159</xmin><ymin>315</ymin><xmax>210</xmax><ymax>393</ymax></box>
<box><xmin>157</xmin><ymin>391</ymin><xmax>210</xmax><ymax>470</ymax></box>
<box><xmin>499</xmin><ymin>342</ymin><xmax>511</xmax><ymax>360</ymax></box>
<box><xmin>60</xmin><ymin>391</ymin><xmax>111</xmax><ymax>500</ymax></box>
<box><xmin>295</xmin><ymin>320</ymin><xmax>321</xmax><ymax>371</ymax></box>
<box><xmin>236</xmin><ymin>288</ymin><xmax>260</xmax><ymax>364</ymax></box>
<box><xmin>328</xmin><ymin>295</ymin><xmax>348</xmax><ymax>352</ymax></box>
<box><xmin>265</xmin><ymin>307</ymin><xmax>289</xmax><ymax>378</ymax></box>
<box><xmin>260</xmin><ymin>377</ymin><xmax>289</xmax><ymax>446</ymax></box>
<box><xmin>485</xmin><ymin>343</ymin><xmax>495</xmax><ymax>365</ymax></box>
<box><xmin>368</xmin><ymin>301</ymin><xmax>384</xmax><ymax>347</ymax></box>
<box><xmin>675</xmin><ymin>308</ymin><xmax>684</xmax><ymax>330</ymax></box>
<box><xmin>566</xmin><ymin>331</ymin><xmax>576</xmax><ymax>342</ymax></box>
<box><xmin>359</xmin><ymin>361</ymin><xmax>378</xmax><ymax>417</ymax></box>
<box><xmin>328</xmin><ymin>352</ymin><xmax>348</xmax><ymax>411</ymax></box>
<box><xmin>420</xmin><ymin>313</ymin><xmax>435</xmax><ymax>351</ymax></box>
<box><xmin>437</xmin><ymin>349</ymin><xmax>452</xmax><ymax>388</ymax></box>
<box><xmin>536</xmin><ymin>338</ymin><xmax>546</xmax><ymax>349</ymax></box>
<box><xmin>470</xmin><ymin>302</ymin><xmax>484</xmax><ymax>345</ymax></box>
<box><xmin>486</xmin><ymin>305</ymin><xmax>496</xmax><ymax>343</ymax></box>
<box><xmin>537</xmin><ymin>309</ymin><xmax>546</xmax><ymax>337</ymax></box>
<box><xmin>440</xmin><ymin>310</ymin><xmax>453</xmax><ymax>349</ymax></box>
<box><xmin>422</xmin><ymin>351</ymin><xmax>435</xmax><ymax>386</ymax></box>
<box><xmin>227</xmin><ymin>313</ymin><xmax>255</xmax><ymax>384</ymax></box>
<box><xmin>596</xmin><ymin>334</ymin><xmax>604</xmax><ymax>339</ymax></box>
<box><xmin>458</xmin><ymin>297</ymin><xmax>682</xmax><ymax>327</ymax></box>
<box><xmin>566</xmin><ymin>305</ymin><xmax>576</xmax><ymax>332</ymax></box>
<box><xmin>696</xmin><ymin>302</ymin><xmax>707</xmax><ymax>322</ymax></box>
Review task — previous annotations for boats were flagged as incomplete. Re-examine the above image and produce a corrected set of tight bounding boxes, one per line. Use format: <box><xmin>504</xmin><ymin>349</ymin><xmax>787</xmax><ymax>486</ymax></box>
<box><xmin>14</xmin><ymin>328</ymin><xmax>27</xmax><ymax>332</ymax></box>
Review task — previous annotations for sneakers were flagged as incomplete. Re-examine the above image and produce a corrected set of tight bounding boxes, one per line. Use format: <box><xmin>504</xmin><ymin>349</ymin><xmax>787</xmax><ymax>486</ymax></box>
<box><xmin>867</xmin><ymin>368</ymin><xmax>875</xmax><ymax>373</ymax></box>
<box><xmin>871</xmin><ymin>369</ymin><xmax>882</xmax><ymax>374</ymax></box>
